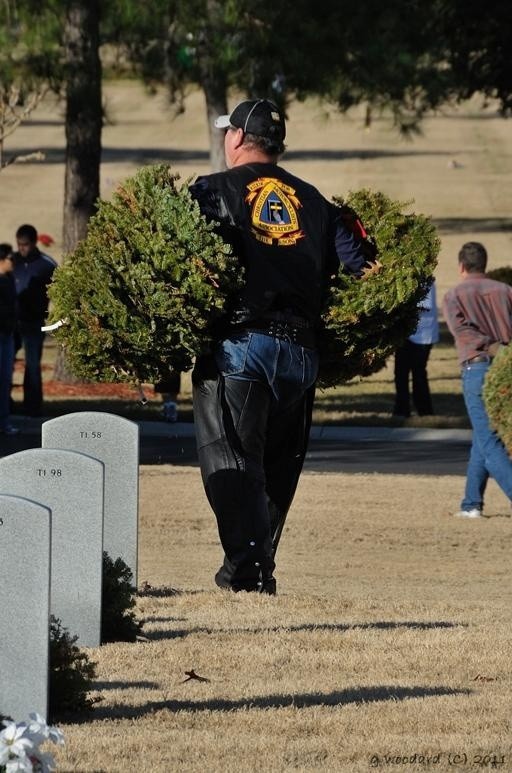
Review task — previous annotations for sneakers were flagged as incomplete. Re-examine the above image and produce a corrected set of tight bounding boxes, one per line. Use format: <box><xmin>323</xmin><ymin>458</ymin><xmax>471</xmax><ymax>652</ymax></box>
<box><xmin>450</xmin><ymin>506</ymin><xmax>483</xmax><ymax>519</ymax></box>
<box><xmin>156</xmin><ymin>402</ymin><xmax>179</xmax><ymax>423</ymax></box>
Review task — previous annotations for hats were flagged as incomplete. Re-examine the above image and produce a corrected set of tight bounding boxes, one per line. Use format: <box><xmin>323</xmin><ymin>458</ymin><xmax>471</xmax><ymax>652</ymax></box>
<box><xmin>212</xmin><ymin>97</ymin><xmax>287</xmax><ymax>144</ymax></box>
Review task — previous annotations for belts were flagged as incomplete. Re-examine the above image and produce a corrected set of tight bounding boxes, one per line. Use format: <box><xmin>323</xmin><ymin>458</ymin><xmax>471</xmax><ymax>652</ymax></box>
<box><xmin>461</xmin><ymin>354</ymin><xmax>494</xmax><ymax>367</ymax></box>
<box><xmin>235</xmin><ymin>311</ymin><xmax>322</xmax><ymax>352</ymax></box>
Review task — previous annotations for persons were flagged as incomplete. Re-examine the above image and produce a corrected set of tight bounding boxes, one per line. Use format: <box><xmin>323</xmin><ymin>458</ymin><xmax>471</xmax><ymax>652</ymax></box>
<box><xmin>10</xmin><ymin>223</ymin><xmax>59</xmax><ymax>420</ymax></box>
<box><xmin>182</xmin><ymin>97</ymin><xmax>382</xmax><ymax>598</ymax></box>
<box><xmin>154</xmin><ymin>373</ymin><xmax>182</xmax><ymax>424</ymax></box>
<box><xmin>0</xmin><ymin>242</ymin><xmax>22</xmax><ymax>436</ymax></box>
<box><xmin>389</xmin><ymin>262</ymin><xmax>440</xmax><ymax>421</ymax></box>
<box><xmin>435</xmin><ymin>241</ymin><xmax>511</xmax><ymax>519</ymax></box>
